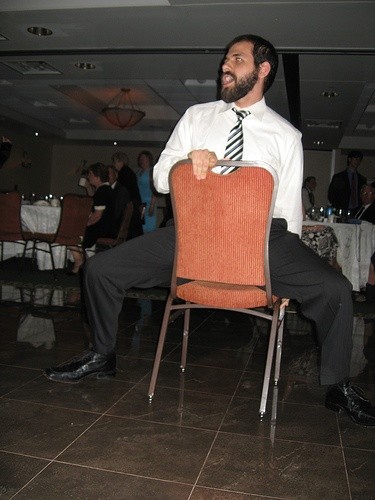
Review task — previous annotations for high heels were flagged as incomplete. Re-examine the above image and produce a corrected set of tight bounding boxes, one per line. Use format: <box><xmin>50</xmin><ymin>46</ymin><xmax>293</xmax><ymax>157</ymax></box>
<box><xmin>67</xmin><ymin>260</ymin><xmax>86</xmax><ymax>276</ymax></box>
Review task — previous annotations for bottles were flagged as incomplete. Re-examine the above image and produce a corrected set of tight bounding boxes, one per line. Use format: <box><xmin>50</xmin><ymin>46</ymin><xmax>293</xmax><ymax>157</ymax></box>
<box><xmin>30</xmin><ymin>192</ymin><xmax>36</xmax><ymax>204</ymax></box>
<box><xmin>21</xmin><ymin>194</ymin><xmax>25</xmax><ymax>201</ymax></box>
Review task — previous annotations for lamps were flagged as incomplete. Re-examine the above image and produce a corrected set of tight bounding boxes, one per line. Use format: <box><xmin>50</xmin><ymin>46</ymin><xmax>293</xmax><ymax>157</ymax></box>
<box><xmin>103</xmin><ymin>87</ymin><xmax>146</xmax><ymax>129</ymax></box>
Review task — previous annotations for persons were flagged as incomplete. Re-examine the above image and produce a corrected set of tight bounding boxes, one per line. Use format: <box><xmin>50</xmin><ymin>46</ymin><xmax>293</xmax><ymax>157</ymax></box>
<box><xmin>302</xmin><ymin>150</ymin><xmax>375</xmax><ymax>224</ymax></box>
<box><xmin>44</xmin><ymin>34</ymin><xmax>375</xmax><ymax>427</ymax></box>
<box><xmin>72</xmin><ymin>150</ymin><xmax>158</xmax><ymax>273</ymax></box>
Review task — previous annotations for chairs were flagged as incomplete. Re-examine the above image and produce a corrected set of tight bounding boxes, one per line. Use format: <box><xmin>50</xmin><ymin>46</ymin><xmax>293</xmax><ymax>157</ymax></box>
<box><xmin>86</xmin><ymin>201</ymin><xmax>136</xmax><ymax>255</ymax></box>
<box><xmin>148</xmin><ymin>158</ymin><xmax>285</xmax><ymax>419</ymax></box>
<box><xmin>0</xmin><ymin>192</ymin><xmax>34</xmax><ymax>274</ymax></box>
<box><xmin>30</xmin><ymin>194</ymin><xmax>94</xmax><ymax>283</ymax></box>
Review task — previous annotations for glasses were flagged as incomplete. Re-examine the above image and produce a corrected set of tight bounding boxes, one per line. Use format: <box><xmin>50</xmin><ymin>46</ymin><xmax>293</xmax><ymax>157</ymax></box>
<box><xmin>361</xmin><ymin>192</ymin><xmax>372</xmax><ymax>195</ymax></box>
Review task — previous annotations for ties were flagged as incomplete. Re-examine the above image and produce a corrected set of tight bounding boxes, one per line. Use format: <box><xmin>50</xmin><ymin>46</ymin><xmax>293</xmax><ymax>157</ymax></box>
<box><xmin>354</xmin><ymin>206</ymin><xmax>364</xmax><ymax>219</ymax></box>
<box><xmin>219</xmin><ymin>107</ymin><xmax>251</xmax><ymax>176</ymax></box>
<box><xmin>350</xmin><ymin>172</ymin><xmax>356</xmax><ymax>209</ymax></box>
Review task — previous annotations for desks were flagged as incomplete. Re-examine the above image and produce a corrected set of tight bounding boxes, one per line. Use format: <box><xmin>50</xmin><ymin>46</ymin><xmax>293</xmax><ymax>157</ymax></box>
<box><xmin>303</xmin><ymin>219</ymin><xmax>375</xmax><ymax>291</ymax></box>
<box><xmin>0</xmin><ymin>204</ymin><xmax>69</xmax><ymax>271</ymax></box>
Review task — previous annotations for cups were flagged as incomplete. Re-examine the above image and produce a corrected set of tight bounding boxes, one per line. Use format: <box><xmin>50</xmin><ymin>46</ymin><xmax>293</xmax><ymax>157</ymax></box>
<box><xmin>79</xmin><ymin>178</ymin><xmax>87</xmax><ymax>186</ymax></box>
<box><xmin>45</xmin><ymin>193</ymin><xmax>64</xmax><ymax>206</ymax></box>
<box><xmin>308</xmin><ymin>204</ymin><xmax>353</xmax><ymax>222</ymax></box>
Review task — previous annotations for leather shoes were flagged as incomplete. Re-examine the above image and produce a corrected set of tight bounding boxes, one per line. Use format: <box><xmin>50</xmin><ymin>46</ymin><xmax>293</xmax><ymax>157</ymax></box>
<box><xmin>324</xmin><ymin>380</ymin><xmax>374</xmax><ymax>427</ymax></box>
<box><xmin>43</xmin><ymin>350</ymin><xmax>117</xmax><ymax>383</ymax></box>
